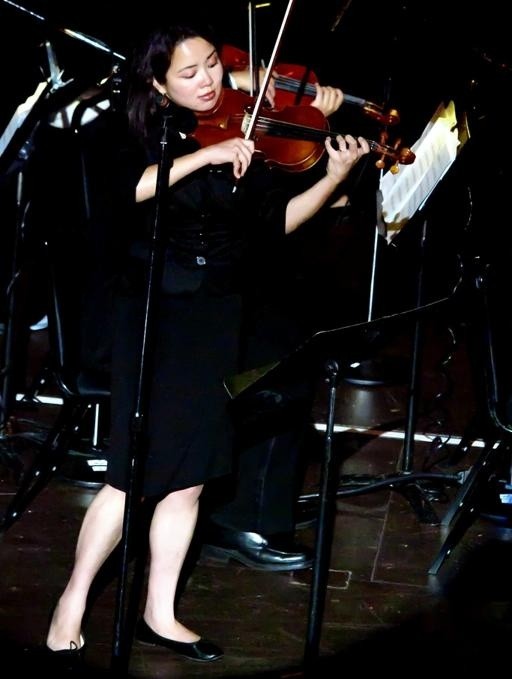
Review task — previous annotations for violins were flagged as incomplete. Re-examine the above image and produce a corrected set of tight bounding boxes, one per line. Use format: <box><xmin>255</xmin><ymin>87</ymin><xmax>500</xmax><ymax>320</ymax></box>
<box><xmin>222</xmin><ymin>45</ymin><xmax>402</xmax><ymax>127</ymax></box>
<box><xmin>189</xmin><ymin>88</ymin><xmax>415</xmax><ymax>174</ymax></box>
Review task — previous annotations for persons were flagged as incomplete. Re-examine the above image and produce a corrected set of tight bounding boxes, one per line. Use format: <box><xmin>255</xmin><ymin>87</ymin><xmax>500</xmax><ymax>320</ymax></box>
<box><xmin>32</xmin><ymin>23</ymin><xmax>373</xmax><ymax>678</ymax></box>
<box><xmin>177</xmin><ymin>147</ymin><xmax>379</xmax><ymax>577</ymax></box>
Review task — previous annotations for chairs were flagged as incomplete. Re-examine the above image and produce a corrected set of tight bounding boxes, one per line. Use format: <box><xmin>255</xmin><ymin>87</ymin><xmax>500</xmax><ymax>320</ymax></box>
<box><xmin>48</xmin><ymin>279</ymin><xmax>114</xmax><ymax>491</ymax></box>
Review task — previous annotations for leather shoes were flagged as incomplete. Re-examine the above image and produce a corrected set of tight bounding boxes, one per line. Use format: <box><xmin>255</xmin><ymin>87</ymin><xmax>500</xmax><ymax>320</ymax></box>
<box><xmin>198</xmin><ymin>529</ymin><xmax>314</xmax><ymax>573</ymax></box>
<box><xmin>134</xmin><ymin>611</ymin><xmax>224</xmax><ymax>663</ymax></box>
<box><xmin>39</xmin><ymin>636</ymin><xmax>89</xmax><ymax>678</ymax></box>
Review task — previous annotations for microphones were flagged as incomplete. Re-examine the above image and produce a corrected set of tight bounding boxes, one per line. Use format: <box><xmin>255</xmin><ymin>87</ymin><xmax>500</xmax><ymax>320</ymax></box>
<box><xmin>147</xmin><ymin>87</ymin><xmax>197</xmax><ymax>134</ymax></box>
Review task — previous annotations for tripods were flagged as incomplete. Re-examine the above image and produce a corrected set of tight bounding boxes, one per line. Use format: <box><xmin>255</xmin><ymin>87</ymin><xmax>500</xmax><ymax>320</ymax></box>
<box><xmin>300</xmin><ymin>213</ymin><xmax>462</xmax><ymax>503</ymax></box>
